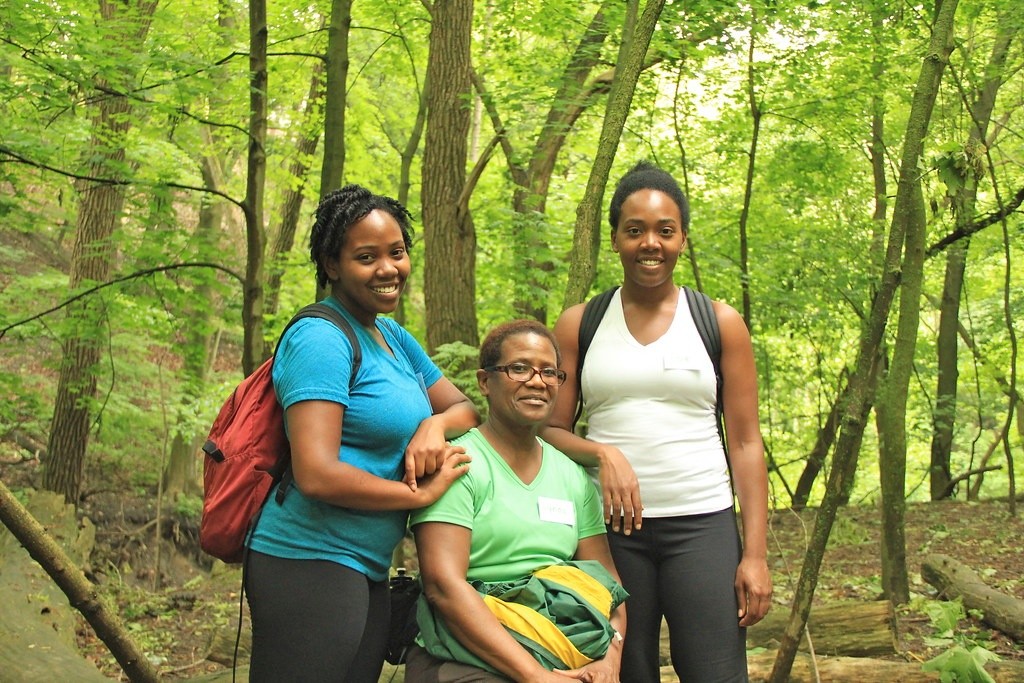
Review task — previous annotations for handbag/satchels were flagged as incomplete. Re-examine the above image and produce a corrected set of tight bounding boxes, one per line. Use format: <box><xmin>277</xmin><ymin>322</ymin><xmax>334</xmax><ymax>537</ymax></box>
<box><xmin>385</xmin><ymin>567</ymin><xmax>421</xmax><ymax>665</ymax></box>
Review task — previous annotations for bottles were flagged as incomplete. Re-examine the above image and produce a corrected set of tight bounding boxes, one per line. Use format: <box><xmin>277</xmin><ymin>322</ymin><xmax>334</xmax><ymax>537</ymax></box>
<box><xmin>386</xmin><ymin>566</ymin><xmax>417</xmax><ymax>665</ymax></box>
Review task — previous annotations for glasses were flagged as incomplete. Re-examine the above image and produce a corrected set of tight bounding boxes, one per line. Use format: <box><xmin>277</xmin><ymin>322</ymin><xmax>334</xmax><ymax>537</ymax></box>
<box><xmin>484</xmin><ymin>362</ymin><xmax>567</xmax><ymax>387</ymax></box>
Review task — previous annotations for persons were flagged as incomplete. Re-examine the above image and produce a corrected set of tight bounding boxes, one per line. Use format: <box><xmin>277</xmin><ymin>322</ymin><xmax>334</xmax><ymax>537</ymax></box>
<box><xmin>404</xmin><ymin>318</ymin><xmax>631</xmax><ymax>683</ymax></box>
<box><xmin>240</xmin><ymin>184</ymin><xmax>482</xmax><ymax>683</ymax></box>
<box><xmin>541</xmin><ymin>163</ymin><xmax>775</xmax><ymax>683</ymax></box>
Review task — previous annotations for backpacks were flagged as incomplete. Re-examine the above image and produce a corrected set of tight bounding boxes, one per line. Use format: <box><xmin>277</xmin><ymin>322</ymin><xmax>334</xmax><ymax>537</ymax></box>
<box><xmin>198</xmin><ymin>303</ymin><xmax>363</xmax><ymax>564</ymax></box>
